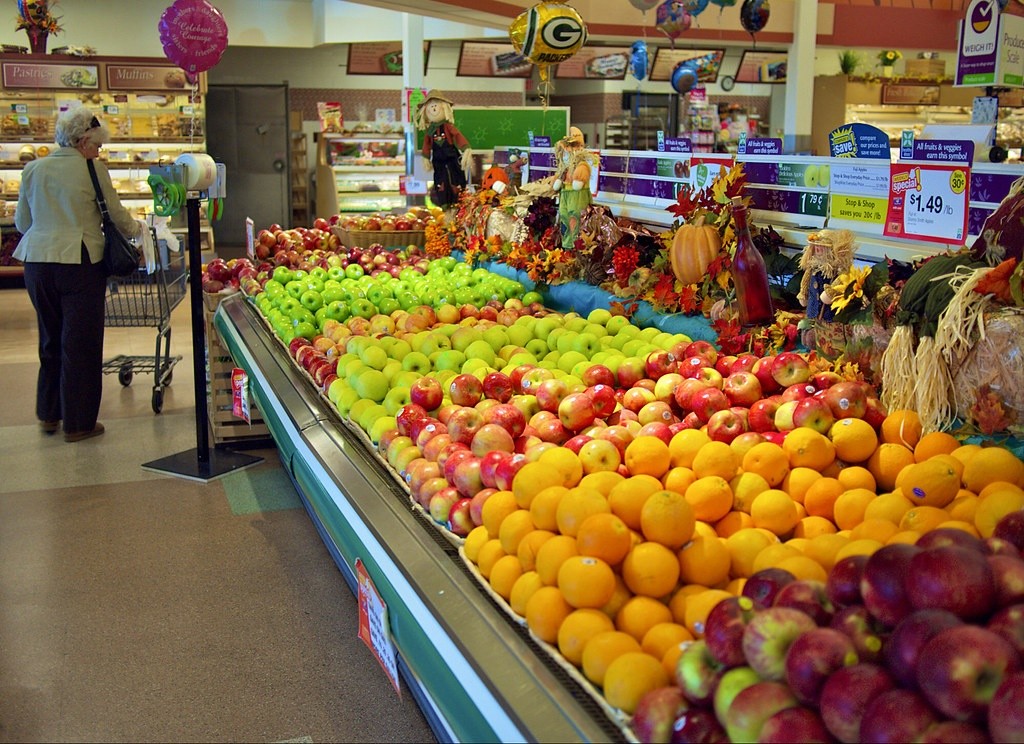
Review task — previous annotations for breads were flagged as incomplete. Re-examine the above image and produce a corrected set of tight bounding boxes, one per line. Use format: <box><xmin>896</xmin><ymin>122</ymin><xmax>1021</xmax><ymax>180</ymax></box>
<box><xmin>60</xmin><ymin>68</ymin><xmax>97</xmax><ymax>87</ymax></box>
<box><xmin>0</xmin><ymin>115</ymin><xmax>50</xmax><ymax>218</ymax></box>
<box><xmin>98</xmin><ymin>154</ymin><xmax>171</xmax><ymax>218</ymax></box>
<box><xmin>111</xmin><ymin>115</ymin><xmax>204</xmax><ymax>137</ymax></box>
<box><xmin>164</xmin><ymin>70</ymin><xmax>187</xmax><ymax>89</ymax></box>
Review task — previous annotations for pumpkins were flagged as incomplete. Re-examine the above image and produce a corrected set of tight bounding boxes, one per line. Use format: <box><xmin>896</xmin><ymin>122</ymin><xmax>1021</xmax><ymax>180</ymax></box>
<box><xmin>671</xmin><ymin>216</ymin><xmax>722</xmax><ymax>285</ymax></box>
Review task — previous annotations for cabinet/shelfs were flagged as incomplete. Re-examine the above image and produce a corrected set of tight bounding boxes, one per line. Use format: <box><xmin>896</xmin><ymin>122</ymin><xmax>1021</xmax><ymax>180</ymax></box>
<box><xmin>0</xmin><ymin>93</ymin><xmax>213</xmax><ymax>279</ymax></box>
<box><xmin>315</xmin><ymin>131</ymin><xmax>408</xmax><ymax>225</ymax></box>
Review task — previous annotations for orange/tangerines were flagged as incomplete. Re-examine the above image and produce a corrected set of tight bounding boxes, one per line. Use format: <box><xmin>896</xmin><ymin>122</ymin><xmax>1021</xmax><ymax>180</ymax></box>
<box><xmin>463</xmin><ymin>408</ymin><xmax>1024</xmax><ymax>712</ymax></box>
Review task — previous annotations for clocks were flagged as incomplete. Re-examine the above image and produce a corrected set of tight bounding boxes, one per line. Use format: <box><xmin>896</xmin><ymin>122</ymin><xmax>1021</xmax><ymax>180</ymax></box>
<box><xmin>721</xmin><ymin>75</ymin><xmax>735</xmax><ymax>90</ymax></box>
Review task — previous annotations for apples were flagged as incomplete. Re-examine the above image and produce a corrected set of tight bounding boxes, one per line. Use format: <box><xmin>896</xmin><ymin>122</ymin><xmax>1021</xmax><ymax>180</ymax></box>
<box><xmin>253</xmin><ymin>218</ymin><xmax>340</xmax><ymax>257</ymax></box>
<box><xmin>203</xmin><ymin>243</ymin><xmax>886</xmax><ymax>533</ymax></box>
<box><xmin>329</xmin><ymin>207</ymin><xmax>447</xmax><ymax>232</ymax></box>
<box><xmin>635</xmin><ymin>511</ymin><xmax>1024</xmax><ymax>744</ymax></box>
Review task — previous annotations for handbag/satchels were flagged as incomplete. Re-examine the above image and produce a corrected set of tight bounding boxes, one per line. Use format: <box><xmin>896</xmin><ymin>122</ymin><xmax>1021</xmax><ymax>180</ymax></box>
<box><xmin>85</xmin><ymin>158</ymin><xmax>142</xmax><ymax>278</ymax></box>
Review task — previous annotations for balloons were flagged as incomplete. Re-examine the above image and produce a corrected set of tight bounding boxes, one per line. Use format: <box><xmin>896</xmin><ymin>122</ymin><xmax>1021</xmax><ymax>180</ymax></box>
<box><xmin>158</xmin><ymin>0</ymin><xmax>229</xmax><ymax>81</ymax></box>
<box><xmin>510</xmin><ymin>0</ymin><xmax>590</xmax><ymax>81</ymax></box>
<box><xmin>629</xmin><ymin>0</ymin><xmax>770</xmax><ymax>91</ymax></box>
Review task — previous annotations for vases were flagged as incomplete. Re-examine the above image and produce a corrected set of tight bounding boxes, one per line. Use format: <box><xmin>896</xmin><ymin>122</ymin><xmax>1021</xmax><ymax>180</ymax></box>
<box><xmin>26</xmin><ymin>30</ymin><xmax>48</xmax><ymax>53</ymax></box>
<box><xmin>880</xmin><ymin>65</ymin><xmax>893</xmax><ymax>79</ymax></box>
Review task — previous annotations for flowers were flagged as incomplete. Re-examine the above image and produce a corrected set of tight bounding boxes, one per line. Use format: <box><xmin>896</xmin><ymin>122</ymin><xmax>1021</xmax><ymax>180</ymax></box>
<box><xmin>14</xmin><ymin>0</ymin><xmax>64</xmax><ymax>37</ymax></box>
<box><xmin>873</xmin><ymin>48</ymin><xmax>903</xmax><ymax>68</ymax></box>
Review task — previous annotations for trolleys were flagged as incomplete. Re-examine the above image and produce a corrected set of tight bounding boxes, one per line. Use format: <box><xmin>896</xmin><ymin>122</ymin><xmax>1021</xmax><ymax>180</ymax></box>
<box><xmin>102</xmin><ymin>226</ymin><xmax>187</xmax><ymax>413</ymax></box>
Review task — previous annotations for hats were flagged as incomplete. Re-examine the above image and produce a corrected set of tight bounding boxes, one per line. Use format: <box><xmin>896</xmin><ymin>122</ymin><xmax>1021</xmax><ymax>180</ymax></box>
<box><xmin>417</xmin><ymin>88</ymin><xmax>454</xmax><ymax>109</ymax></box>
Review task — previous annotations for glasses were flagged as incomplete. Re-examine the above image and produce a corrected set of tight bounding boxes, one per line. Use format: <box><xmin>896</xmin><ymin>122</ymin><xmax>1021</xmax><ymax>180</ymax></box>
<box><xmin>75</xmin><ymin>115</ymin><xmax>101</xmax><ymax>144</ymax></box>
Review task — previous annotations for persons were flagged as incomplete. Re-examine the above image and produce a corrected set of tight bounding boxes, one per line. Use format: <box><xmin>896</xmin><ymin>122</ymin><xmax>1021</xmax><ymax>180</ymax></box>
<box><xmin>414</xmin><ymin>88</ymin><xmax>476</xmax><ymax>208</ymax></box>
<box><xmin>12</xmin><ymin>109</ymin><xmax>144</xmax><ymax>444</ymax></box>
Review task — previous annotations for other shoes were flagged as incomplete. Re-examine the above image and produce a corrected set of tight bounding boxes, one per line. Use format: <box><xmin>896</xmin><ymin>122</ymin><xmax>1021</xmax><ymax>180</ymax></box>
<box><xmin>39</xmin><ymin>421</ymin><xmax>61</xmax><ymax>435</ymax></box>
<box><xmin>62</xmin><ymin>422</ymin><xmax>105</xmax><ymax>442</ymax></box>
